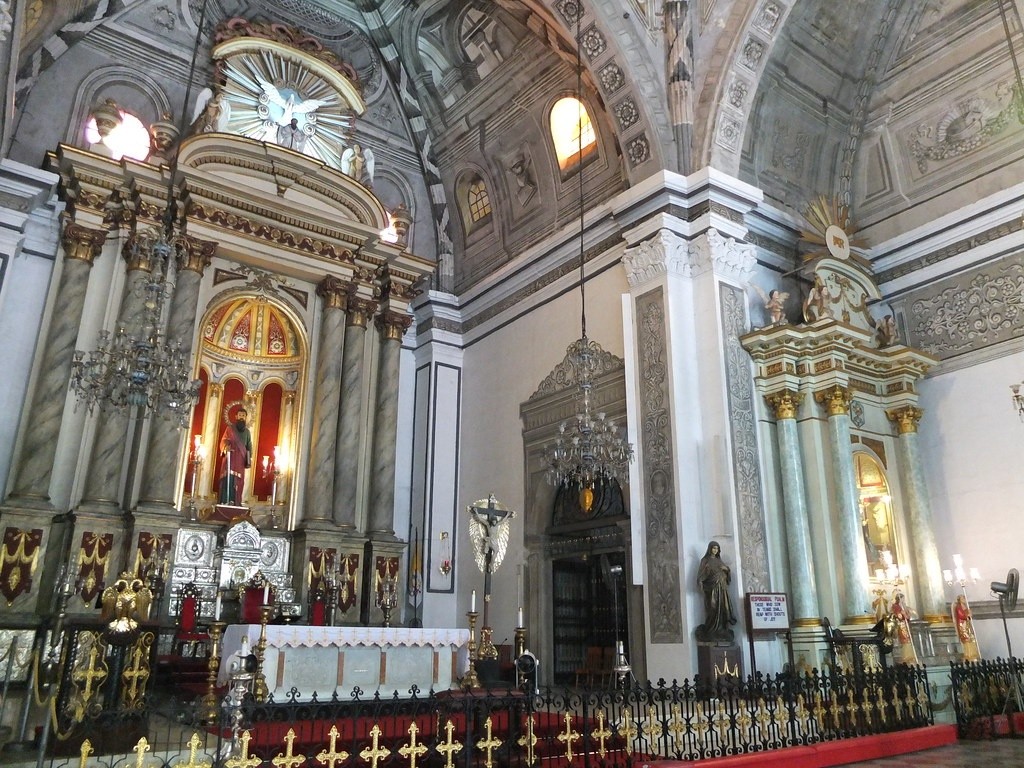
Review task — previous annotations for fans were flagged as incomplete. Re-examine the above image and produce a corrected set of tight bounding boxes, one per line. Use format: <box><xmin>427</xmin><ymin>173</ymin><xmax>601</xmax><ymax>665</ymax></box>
<box><xmin>600</xmin><ymin>553</ymin><xmax>637</xmax><ymax>692</ymax></box>
<box><xmin>990</xmin><ymin>568</ymin><xmax>1019</xmax><ymax>663</ymax></box>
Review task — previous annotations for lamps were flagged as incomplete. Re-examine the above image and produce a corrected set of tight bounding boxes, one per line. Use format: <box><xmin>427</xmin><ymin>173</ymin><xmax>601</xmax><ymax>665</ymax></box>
<box><xmin>539</xmin><ymin>0</ymin><xmax>636</xmax><ymax>513</ymax></box>
<box><xmin>261</xmin><ymin>438</ymin><xmax>294</xmax><ymax>479</ymax></box>
<box><xmin>190</xmin><ymin>434</ymin><xmax>208</xmax><ymax>465</ymax></box>
<box><xmin>144</xmin><ymin>540</ymin><xmax>172</xmax><ymax>621</ymax></box>
<box><xmin>68</xmin><ymin>0</ymin><xmax>209</xmax><ymax>430</ymax></box>
<box><xmin>47</xmin><ymin>552</ymin><xmax>85</xmax><ymax>657</ymax></box>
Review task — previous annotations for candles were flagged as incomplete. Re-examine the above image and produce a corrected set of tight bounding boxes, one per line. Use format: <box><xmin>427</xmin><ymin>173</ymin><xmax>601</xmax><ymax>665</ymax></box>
<box><xmin>518</xmin><ymin>607</ymin><xmax>523</xmax><ymax>627</ymax></box>
<box><xmin>333</xmin><ymin>579</ymin><xmax>335</xmax><ymax>587</ymax></box>
<box><xmin>471</xmin><ymin>590</ymin><xmax>475</xmax><ymax>613</ymax></box>
<box><xmin>215</xmin><ymin>592</ymin><xmax>222</xmax><ymax>621</ymax></box>
<box><xmin>444</xmin><ymin>560</ymin><xmax>449</xmax><ymax>567</ymax></box>
<box><xmin>263</xmin><ymin>582</ymin><xmax>269</xmax><ymax>605</ymax></box>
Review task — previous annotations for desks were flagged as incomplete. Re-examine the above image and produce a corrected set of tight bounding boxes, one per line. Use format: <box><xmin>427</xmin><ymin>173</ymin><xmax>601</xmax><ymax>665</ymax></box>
<box><xmin>217</xmin><ymin>625</ymin><xmax>471</xmax><ymax>704</ymax></box>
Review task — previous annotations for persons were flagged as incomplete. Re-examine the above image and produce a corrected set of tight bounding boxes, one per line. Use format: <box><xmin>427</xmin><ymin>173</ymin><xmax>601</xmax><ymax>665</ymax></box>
<box><xmin>890</xmin><ymin>593</ymin><xmax>918</xmax><ymax>667</ymax></box>
<box><xmin>954</xmin><ymin>594</ymin><xmax>980</xmax><ymax>659</ymax></box>
<box><xmin>220</xmin><ymin>409</ymin><xmax>253</xmax><ymax>505</ymax></box>
<box><xmin>697</xmin><ymin>541</ymin><xmax>737</xmax><ymax>635</ymax></box>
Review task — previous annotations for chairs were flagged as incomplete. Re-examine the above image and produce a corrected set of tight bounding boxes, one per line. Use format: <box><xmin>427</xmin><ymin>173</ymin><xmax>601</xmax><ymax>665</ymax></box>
<box><xmin>306</xmin><ymin>577</ymin><xmax>332</xmax><ymax>626</ymax></box>
<box><xmin>238</xmin><ymin>570</ymin><xmax>279</xmax><ymax>624</ymax></box>
<box><xmin>167</xmin><ymin>581</ymin><xmax>210</xmax><ymax>684</ymax></box>
